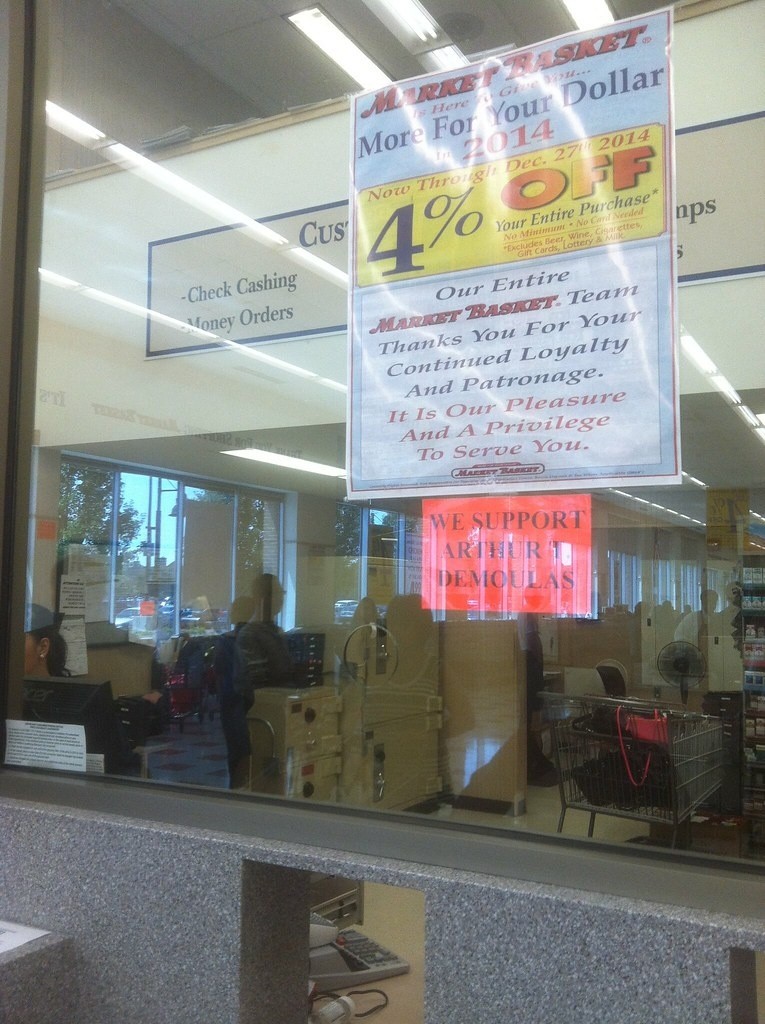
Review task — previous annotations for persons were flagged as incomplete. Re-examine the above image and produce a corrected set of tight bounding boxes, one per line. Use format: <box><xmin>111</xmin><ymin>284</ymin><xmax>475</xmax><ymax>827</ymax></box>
<box><xmin>633</xmin><ymin>581</ymin><xmax>744</xmax><ymax>693</ymax></box>
<box><xmin>516</xmin><ymin>585</ymin><xmax>555</xmax><ymax>785</ymax></box>
<box><xmin>233</xmin><ymin>574</ymin><xmax>295</xmax><ymax>712</ymax></box>
<box><xmin>23</xmin><ymin>603</ymin><xmax>130</xmax><ymax>773</ymax></box>
<box><xmin>209</xmin><ymin>597</ymin><xmax>256</xmax><ymax>789</ymax></box>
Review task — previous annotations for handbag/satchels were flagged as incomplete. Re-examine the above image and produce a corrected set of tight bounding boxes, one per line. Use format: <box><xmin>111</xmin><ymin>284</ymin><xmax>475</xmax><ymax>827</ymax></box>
<box><xmin>565</xmin><ymin>715</ymin><xmax>691</xmax><ymax>813</ymax></box>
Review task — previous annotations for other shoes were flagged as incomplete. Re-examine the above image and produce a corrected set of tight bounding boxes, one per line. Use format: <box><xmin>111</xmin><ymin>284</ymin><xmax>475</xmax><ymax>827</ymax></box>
<box><xmin>528</xmin><ymin>769</ymin><xmax>568</xmax><ymax>788</ymax></box>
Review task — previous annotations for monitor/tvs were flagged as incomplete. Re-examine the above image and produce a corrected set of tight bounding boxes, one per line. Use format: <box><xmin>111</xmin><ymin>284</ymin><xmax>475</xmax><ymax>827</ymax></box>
<box><xmin>21</xmin><ymin>673</ymin><xmax>140</xmax><ymax>779</ymax></box>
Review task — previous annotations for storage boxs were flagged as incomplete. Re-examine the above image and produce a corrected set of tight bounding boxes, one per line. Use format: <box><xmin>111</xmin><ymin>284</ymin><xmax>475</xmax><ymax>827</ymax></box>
<box><xmin>240</xmin><ymin>688</ymin><xmax>343</xmax><ymax>804</ymax></box>
<box><xmin>296</xmin><ymin>557</ymin><xmax>443</xmax><ymax>815</ymax></box>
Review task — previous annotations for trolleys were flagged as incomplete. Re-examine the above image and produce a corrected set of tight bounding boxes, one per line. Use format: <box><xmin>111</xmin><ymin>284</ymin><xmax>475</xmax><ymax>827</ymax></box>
<box><xmin>535</xmin><ymin>690</ymin><xmax>726</xmax><ymax>848</ymax></box>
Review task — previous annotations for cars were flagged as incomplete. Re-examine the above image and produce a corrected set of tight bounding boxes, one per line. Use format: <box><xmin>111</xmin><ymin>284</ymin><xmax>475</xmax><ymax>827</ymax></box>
<box><xmin>115</xmin><ymin>596</ymin><xmax>227</xmax><ymax>634</ymax></box>
<box><xmin>334</xmin><ymin>600</ymin><xmax>387</xmax><ymax>623</ymax></box>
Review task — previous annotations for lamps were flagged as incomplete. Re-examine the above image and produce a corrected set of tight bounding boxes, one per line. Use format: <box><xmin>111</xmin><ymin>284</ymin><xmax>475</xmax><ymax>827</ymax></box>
<box><xmin>276</xmin><ymin>0</ymin><xmax>639</xmax><ymax>87</ymax></box>
<box><xmin>218</xmin><ymin>445</ymin><xmax>347</xmax><ymax>485</ymax></box>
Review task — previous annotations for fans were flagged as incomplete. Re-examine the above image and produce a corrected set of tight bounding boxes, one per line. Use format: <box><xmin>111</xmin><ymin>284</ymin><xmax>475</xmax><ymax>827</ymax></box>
<box><xmin>655</xmin><ymin>641</ymin><xmax>707</xmax><ymax>709</ymax></box>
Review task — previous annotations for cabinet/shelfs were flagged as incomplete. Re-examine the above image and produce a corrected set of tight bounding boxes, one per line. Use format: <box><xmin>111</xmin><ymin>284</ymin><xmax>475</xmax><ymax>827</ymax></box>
<box><xmin>734</xmin><ymin>549</ymin><xmax>765</xmax><ymax>859</ymax></box>
